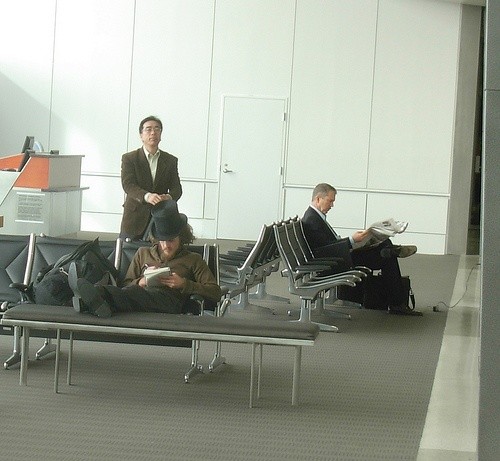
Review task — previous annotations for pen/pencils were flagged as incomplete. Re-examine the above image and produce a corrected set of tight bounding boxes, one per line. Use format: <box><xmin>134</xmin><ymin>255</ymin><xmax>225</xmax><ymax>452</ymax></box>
<box><xmin>145</xmin><ymin>264</ymin><xmax>149</xmax><ymax>268</ymax></box>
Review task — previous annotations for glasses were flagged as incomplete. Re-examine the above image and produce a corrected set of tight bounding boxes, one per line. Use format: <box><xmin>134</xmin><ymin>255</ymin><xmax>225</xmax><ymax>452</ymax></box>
<box><xmin>141</xmin><ymin>128</ymin><xmax>162</xmax><ymax>133</ymax></box>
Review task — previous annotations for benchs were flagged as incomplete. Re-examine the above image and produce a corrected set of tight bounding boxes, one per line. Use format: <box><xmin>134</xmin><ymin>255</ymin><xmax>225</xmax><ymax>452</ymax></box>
<box><xmin>0</xmin><ymin>232</ymin><xmax>227</xmax><ymax>384</ymax></box>
<box><xmin>0</xmin><ymin>304</ymin><xmax>320</xmax><ymax>408</ymax></box>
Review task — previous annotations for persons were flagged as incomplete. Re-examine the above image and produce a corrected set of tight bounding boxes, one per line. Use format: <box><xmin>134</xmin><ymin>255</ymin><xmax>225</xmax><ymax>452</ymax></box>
<box><xmin>68</xmin><ymin>199</ymin><xmax>222</xmax><ymax>318</ymax></box>
<box><xmin>119</xmin><ymin>116</ymin><xmax>183</xmax><ymax>242</ymax></box>
<box><xmin>301</xmin><ymin>183</ymin><xmax>423</xmax><ymax>316</ymax></box>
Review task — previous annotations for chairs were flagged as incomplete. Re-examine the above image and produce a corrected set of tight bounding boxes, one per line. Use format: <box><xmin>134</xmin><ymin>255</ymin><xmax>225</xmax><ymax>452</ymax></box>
<box><xmin>218</xmin><ymin>215</ymin><xmax>373</xmax><ymax>333</ymax></box>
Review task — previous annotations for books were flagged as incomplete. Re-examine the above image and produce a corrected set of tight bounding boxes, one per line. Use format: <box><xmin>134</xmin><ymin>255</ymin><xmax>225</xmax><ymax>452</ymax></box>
<box><xmin>144</xmin><ymin>266</ymin><xmax>172</xmax><ymax>287</ymax></box>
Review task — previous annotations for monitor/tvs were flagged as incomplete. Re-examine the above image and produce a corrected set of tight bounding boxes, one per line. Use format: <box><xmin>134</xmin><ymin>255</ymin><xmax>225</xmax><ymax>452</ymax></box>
<box><xmin>18</xmin><ymin>136</ymin><xmax>34</xmax><ymax>172</ymax></box>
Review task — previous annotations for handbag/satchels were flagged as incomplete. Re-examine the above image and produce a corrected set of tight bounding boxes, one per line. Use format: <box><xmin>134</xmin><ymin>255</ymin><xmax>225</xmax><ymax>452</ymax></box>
<box><xmin>361</xmin><ymin>272</ymin><xmax>416</xmax><ymax>311</ymax></box>
<box><xmin>28</xmin><ymin>238</ymin><xmax>118</xmax><ymax>305</ymax></box>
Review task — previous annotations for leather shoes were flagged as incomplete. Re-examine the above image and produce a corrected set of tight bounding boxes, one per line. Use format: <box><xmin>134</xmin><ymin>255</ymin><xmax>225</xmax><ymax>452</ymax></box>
<box><xmin>389</xmin><ymin>304</ymin><xmax>422</xmax><ymax>315</ymax></box>
<box><xmin>381</xmin><ymin>244</ymin><xmax>418</xmax><ymax>258</ymax></box>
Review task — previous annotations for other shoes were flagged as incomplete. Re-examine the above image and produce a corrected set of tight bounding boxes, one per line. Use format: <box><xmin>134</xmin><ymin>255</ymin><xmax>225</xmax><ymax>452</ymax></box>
<box><xmin>72</xmin><ymin>278</ymin><xmax>112</xmax><ymax>318</ymax></box>
<box><xmin>67</xmin><ymin>263</ymin><xmax>87</xmax><ymax>312</ymax></box>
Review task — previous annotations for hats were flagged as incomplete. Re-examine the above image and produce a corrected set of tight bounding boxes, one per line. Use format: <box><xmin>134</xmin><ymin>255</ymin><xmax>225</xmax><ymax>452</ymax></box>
<box><xmin>149</xmin><ymin>200</ymin><xmax>188</xmax><ymax>241</ymax></box>
